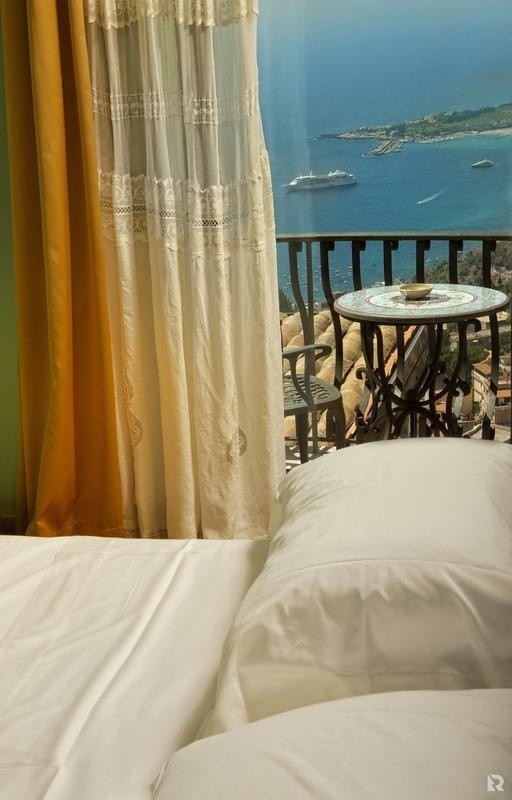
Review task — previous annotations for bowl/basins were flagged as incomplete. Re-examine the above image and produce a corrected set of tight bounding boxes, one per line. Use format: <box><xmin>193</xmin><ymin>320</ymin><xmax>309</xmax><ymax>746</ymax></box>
<box><xmin>399</xmin><ymin>282</ymin><xmax>434</xmax><ymax>301</ymax></box>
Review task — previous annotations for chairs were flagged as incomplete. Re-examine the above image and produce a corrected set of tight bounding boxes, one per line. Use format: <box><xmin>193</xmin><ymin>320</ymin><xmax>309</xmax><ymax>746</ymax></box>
<box><xmin>280</xmin><ymin>320</ymin><xmax>346</xmax><ymax>472</ymax></box>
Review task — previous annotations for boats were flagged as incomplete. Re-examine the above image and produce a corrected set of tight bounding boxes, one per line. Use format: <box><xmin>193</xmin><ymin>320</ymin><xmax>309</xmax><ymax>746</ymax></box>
<box><xmin>472</xmin><ymin>159</ymin><xmax>494</xmax><ymax>168</ymax></box>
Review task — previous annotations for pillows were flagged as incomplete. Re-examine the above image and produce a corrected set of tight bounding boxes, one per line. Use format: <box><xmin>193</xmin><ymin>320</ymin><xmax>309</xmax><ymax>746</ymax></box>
<box><xmin>148</xmin><ymin>688</ymin><xmax>512</xmax><ymax>800</ymax></box>
<box><xmin>199</xmin><ymin>434</ymin><xmax>512</xmax><ymax>740</ymax></box>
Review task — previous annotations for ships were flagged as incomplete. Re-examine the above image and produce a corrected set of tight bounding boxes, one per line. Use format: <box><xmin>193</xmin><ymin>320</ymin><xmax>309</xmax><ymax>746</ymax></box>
<box><xmin>281</xmin><ymin>167</ymin><xmax>357</xmax><ymax>191</ymax></box>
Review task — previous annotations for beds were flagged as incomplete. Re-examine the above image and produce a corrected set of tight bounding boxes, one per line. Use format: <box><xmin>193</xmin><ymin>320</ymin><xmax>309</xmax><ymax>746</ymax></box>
<box><xmin>0</xmin><ymin>532</ymin><xmax>270</xmax><ymax>799</ymax></box>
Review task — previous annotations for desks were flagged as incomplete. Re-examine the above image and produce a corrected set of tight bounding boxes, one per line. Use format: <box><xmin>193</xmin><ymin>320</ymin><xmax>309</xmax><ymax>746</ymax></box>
<box><xmin>331</xmin><ymin>283</ymin><xmax>511</xmax><ymax>440</ymax></box>
